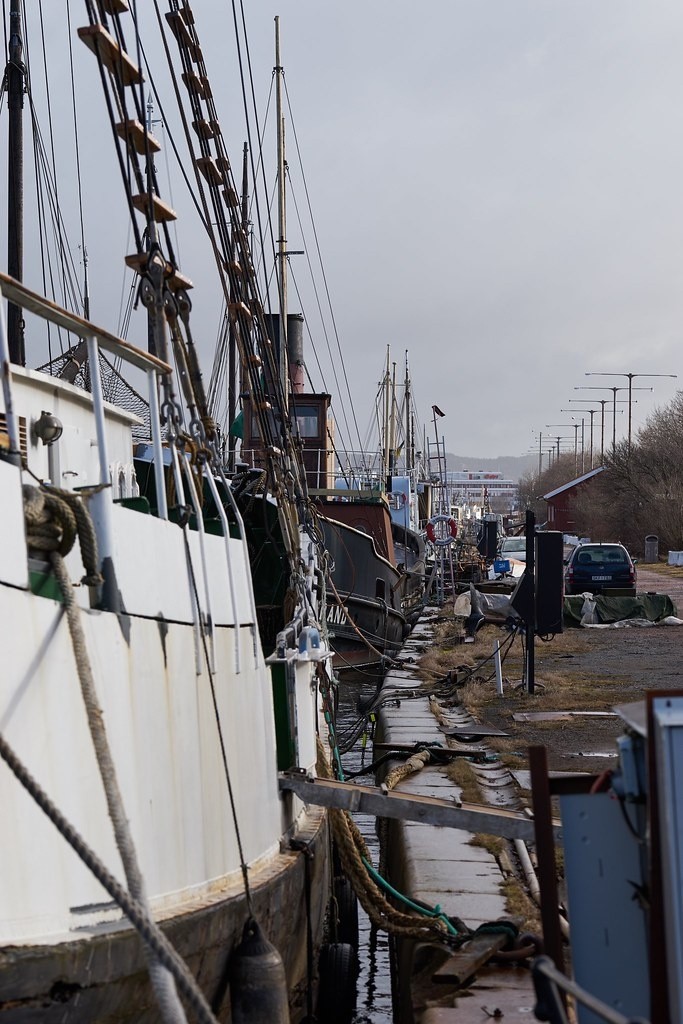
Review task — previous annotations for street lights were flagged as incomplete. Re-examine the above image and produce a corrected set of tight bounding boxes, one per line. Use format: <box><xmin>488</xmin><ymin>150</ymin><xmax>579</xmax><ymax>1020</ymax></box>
<box><xmin>521</xmin><ymin>372</ymin><xmax>677</xmax><ymax>479</ymax></box>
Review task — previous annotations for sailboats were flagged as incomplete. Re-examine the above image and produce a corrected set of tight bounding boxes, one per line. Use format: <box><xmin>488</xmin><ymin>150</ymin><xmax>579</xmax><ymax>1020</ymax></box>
<box><xmin>0</xmin><ymin>1</ymin><xmax>464</xmax><ymax>1024</ymax></box>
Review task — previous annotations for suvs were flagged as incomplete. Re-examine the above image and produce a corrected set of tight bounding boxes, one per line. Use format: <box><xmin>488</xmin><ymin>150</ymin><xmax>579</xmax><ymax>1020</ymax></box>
<box><xmin>562</xmin><ymin>541</ymin><xmax>638</xmax><ymax>598</ymax></box>
<box><xmin>499</xmin><ymin>536</ymin><xmax>535</xmax><ymax>564</ymax></box>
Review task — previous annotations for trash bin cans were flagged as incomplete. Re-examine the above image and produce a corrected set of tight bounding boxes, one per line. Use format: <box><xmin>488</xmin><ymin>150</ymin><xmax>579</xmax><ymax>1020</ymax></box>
<box><xmin>644</xmin><ymin>535</ymin><xmax>659</xmax><ymax>564</ymax></box>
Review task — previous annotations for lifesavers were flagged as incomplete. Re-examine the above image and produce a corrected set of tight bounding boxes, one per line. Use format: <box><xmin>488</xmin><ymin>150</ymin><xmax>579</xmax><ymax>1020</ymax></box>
<box><xmin>387</xmin><ymin>490</ymin><xmax>407</xmax><ymax>511</ymax></box>
<box><xmin>426</xmin><ymin>515</ymin><xmax>458</xmax><ymax>546</ymax></box>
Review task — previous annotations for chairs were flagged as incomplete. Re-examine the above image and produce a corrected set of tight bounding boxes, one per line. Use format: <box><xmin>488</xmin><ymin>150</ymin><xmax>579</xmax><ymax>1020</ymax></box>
<box><xmin>579</xmin><ymin>553</ymin><xmax>593</xmax><ymax>562</ymax></box>
<box><xmin>608</xmin><ymin>552</ymin><xmax>621</xmax><ymax>562</ymax></box>
